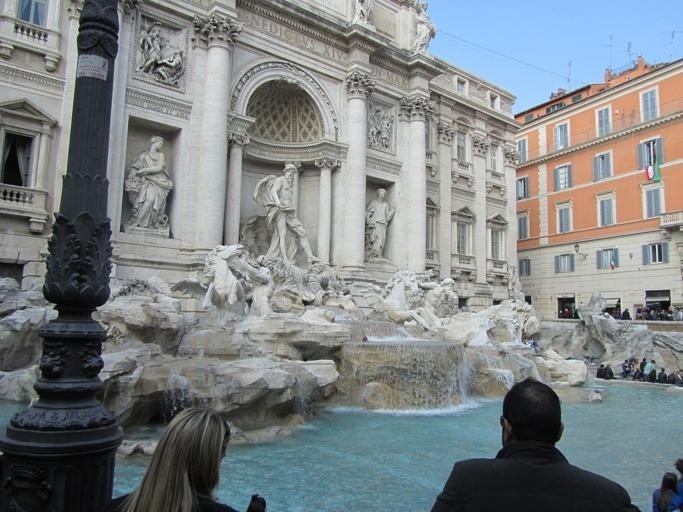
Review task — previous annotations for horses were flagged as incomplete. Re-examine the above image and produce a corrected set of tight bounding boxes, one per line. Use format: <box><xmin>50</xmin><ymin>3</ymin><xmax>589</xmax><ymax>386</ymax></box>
<box><xmin>200</xmin><ymin>243</ymin><xmax>246</xmax><ymax>309</ymax></box>
<box><xmin>377</xmin><ymin>269</ymin><xmax>442</xmax><ymax>330</ymax></box>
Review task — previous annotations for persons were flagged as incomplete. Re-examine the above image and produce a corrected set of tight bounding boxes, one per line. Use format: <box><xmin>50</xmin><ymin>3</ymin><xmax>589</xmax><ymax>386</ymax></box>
<box><xmin>141</xmin><ymin>21</ymin><xmax>163</xmax><ymax>75</ymax></box>
<box><xmin>673</xmin><ymin>458</ymin><xmax>683</xmax><ymax>498</ymax></box>
<box><xmin>112</xmin><ymin>406</ymin><xmax>242</xmax><ymax>511</ymax></box>
<box><xmin>125</xmin><ymin>134</ymin><xmax>173</xmax><ymax>230</ymax></box>
<box><xmin>652</xmin><ymin>472</ymin><xmax>683</xmax><ymax>512</ymax></box>
<box><xmin>363</xmin><ymin>188</ymin><xmax>396</xmax><ymax>258</ymax></box>
<box><xmin>559</xmin><ymin>305</ymin><xmax>683</xmax><ymax>321</ymax></box>
<box><xmin>236</xmin><ymin>259</ymin><xmax>275</xmax><ymax>320</ymax></box>
<box><xmin>596</xmin><ymin>364</ymin><xmax>605</xmax><ymax>378</ymax></box>
<box><xmin>412</xmin><ymin>1</ymin><xmax>437</xmax><ymax>56</ymax></box>
<box><xmin>603</xmin><ymin>364</ymin><xmax>618</xmax><ymax>380</ymax></box>
<box><xmin>622</xmin><ymin>357</ymin><xmax>683</xmax><ymax>386</ymax></box>
<box><xmin>419</xmin><ymin>278</ymin><xmax>455</xmax><ymax>313</ymax></box>
<box><xmin>158</xmin><ymin>47</ymin><xmax>185</xmax><ymax>83</ymax></box>
<box><xmin>529</xmin><ymin>337</ymin><xmax>539</xmax><ymax>353</ymax></box>
<box><xmin>428</xmin><ymin>376</ymin><xmax>641</xmax><ymax>511</ymax></box>
<box><xmin>268</xmin><ymin>163</ymin><xmax>322</xmax><ymax>265</ymax></box>
<box><xmin>245</xmin><ymin>493</ymin><xmax>266</xmax><ymax>511</ymax></box>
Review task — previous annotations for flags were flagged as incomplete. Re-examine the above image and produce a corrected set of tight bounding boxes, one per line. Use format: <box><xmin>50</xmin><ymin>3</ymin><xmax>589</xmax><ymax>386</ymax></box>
<box><xmin>644</xmin><ymin>164</ymin><xmax>661</xmax><ymax>183</ymax></box>
